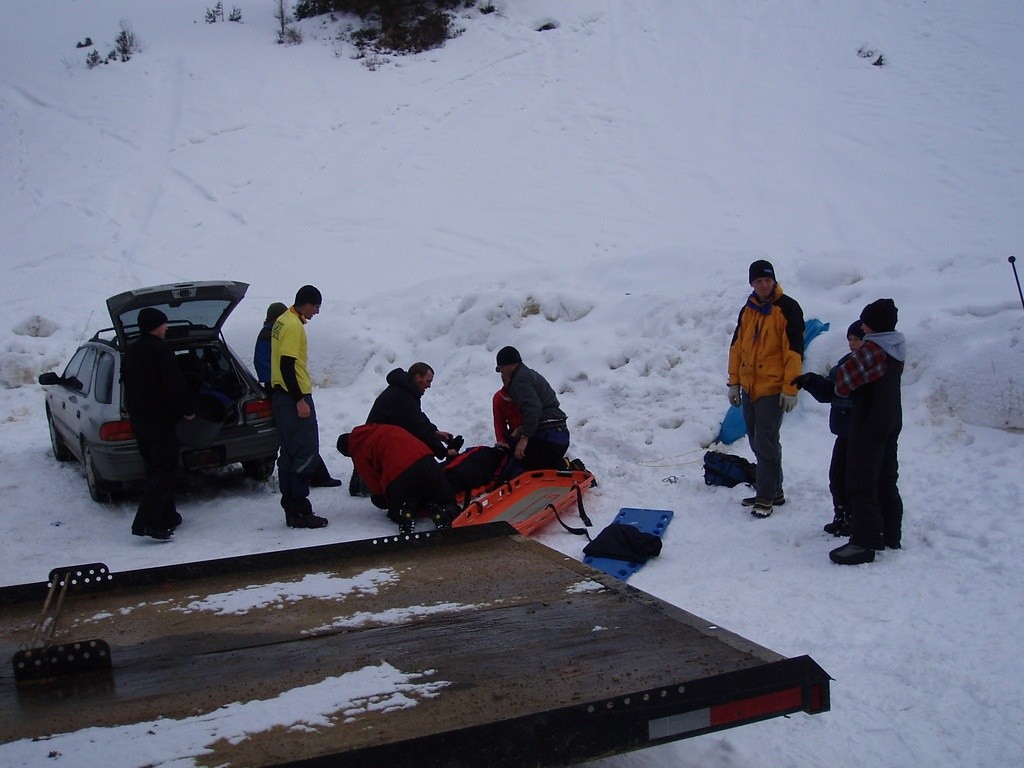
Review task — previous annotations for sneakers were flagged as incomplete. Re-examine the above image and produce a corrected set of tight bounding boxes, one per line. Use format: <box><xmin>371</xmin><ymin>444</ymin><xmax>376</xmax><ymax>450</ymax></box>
<box><xmin>741</xmin><ymin>495</ymin><xmax>785</xmax><ymax>506</ymax></box>
<box><xmin>751</xmin><ymin>498</ymin><xmax>773</xmax><ymax>517</ymax></box>
<box><xmin>569</xmin><ymin>458</ymin><xmax>597</xmax><ymax>487</ymax></box>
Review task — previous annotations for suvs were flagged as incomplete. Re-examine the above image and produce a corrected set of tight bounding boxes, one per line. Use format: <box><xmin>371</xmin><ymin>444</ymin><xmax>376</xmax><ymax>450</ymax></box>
<box><xmin>38</xmin><ymin>280</ymin><xmax>278</xmax><ymax>503</ymax></box>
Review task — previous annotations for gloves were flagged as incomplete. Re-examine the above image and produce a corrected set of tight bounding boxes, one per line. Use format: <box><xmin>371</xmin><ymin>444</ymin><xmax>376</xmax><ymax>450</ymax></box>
<box><xmin>779</xmin><ymin>393</ymin><xmax>798</xmax><ymax>413</ymax></box>
<box><xmin>728</xmin><ymin>385</ymin><xmax>741</xmax><ymax>407</ymax></box>
<box><xmin>790</xmin><ymin>375</ymin><xmax>809</xmax><ymax>390</ymax></box>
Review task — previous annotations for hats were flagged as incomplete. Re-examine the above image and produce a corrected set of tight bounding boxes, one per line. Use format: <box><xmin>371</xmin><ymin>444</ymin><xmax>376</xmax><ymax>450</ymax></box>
<box><xmin>496</xmin><ymin>346</ymin><xmax>522</xmax><ymax>366</ymax></box>
<box><xmin>847</xmin><ymin>320</ymin><xmax>865</xmax><ymax>341</ymax></box>
<box><xmin>295</xmin><ymin>285</ymin><xmax>322</xmax><ymax>306</ymax></box>
<box><xmin>749</xmin><ymin>260</ymin><xmax>774</xmax><ymax>283</ymax></box>
<box><xmin>860</xmin><ymin>299</ymin><xmax>898</xmax><ymax>332</ymax></box>
<box><xmin>137</xmin><ymin>308</ymin><xmax>168</xmax><ymax>332</ymax></box>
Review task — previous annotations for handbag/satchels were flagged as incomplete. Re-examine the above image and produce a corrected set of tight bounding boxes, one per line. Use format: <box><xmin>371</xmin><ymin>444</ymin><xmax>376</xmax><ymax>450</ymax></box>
<box><xmin>703</xmin><ymin>451</ymin><xmax>750</xmax><ymax>487</ymax></box>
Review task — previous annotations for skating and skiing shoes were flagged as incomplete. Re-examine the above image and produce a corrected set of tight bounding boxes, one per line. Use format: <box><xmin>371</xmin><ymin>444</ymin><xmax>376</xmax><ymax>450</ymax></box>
<box><xmin>563</xmin><ymin>457</ymin><xmax>570</xmax><ymax>468</ymax></box>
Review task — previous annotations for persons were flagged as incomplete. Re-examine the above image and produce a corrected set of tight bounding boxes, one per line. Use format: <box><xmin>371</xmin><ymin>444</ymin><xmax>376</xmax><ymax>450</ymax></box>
<box><xmin>253</xmin><ymin>302</ymin><xmax>343</xmax><ymax>487</ymax></box>
<box><xmin>789</xmin><ymin>320</ymin><xmax>866</xmax><ymax>536</ymax></box>
<box><xmin>828</xmin><ymin>298</ymin><xmax>907</xmax><ymax>566</ymax></box>
<box><xmin>725</xmin><ymin>260</ymin><xmax>806</xmax><ymax>520</ymax></box>
<box><xmin>493</xmin><ymin>345</ymin><xmax>599</xmax><ymax>489</ymax></box>
<box><xmin>337</xmin><ymin>421</ymin><xmax>451</xmax><ymax>535</ymax></box>
<box><xmin>271</xmin><ymin>285</ymin><xmax>330</xmax><ymax>529</ymax></box>
<box><xmin>121</xmin><ymin>308</ymin><xmax>196</xmax><ymax>540</ymax></box>
<box><xmin>348</xmin><ymin>362</ymin><xmax>458</xmax><ymax>498</ymax></box>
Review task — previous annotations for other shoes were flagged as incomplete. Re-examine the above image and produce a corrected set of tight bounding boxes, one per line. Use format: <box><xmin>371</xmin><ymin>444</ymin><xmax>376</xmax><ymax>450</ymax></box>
<box><xmin>823</xmin><ymin>514</ymin><xmax>851</xmax><ymax>536</ymax></box>
<box><xmin>874</xmin><ymin>533</ymin><xmax>885</xmax><ymax>549</ymax></box>
<box><xmin>398</xmin><ymin>498</ymin><xmax>418</xmax><ymax>535</ymax></box>
<box><xmin>431</xmin><ymin>505</ymin><xmax>453</xmax><ymax>530</ymax></box>
<box><xmin>311</xmin><ymin>477</ymin><xmax>342</xmax><ymax>487</ymax></box>
<box><xmin>162</xmin><ymin>511</ymin><xmax>182</xmax><ymax>526</ymax></box>
<box><xmin>830</xmin><ymin>543</ymin><xmax>875</xmax><ymax>565</ymax></box>
<box><xmin>131</xmin><ymin>521</ymin><xmax>173</xmax><ymax>541</ymax></box>
<box><xmin>286</xmin><ymin>513</ymin><xmax>328</xmax><ymax>529</ymax></box>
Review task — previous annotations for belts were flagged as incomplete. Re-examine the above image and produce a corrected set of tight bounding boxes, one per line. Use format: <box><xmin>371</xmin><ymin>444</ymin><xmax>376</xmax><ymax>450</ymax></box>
<box><xmin>538</xmin><ymin>421</ymin><xmax>566</xmax><ymax>430</ymax></box>
